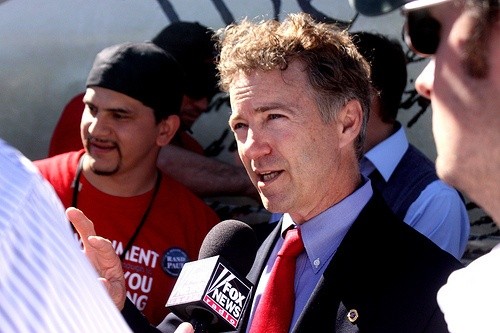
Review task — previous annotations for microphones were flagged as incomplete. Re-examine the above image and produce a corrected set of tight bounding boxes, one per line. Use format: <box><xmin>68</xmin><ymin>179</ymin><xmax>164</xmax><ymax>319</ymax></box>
<box><xmin>165</xmin><ymin>219</ymin><xmax>258</xmax><ymax>333</ymax></box>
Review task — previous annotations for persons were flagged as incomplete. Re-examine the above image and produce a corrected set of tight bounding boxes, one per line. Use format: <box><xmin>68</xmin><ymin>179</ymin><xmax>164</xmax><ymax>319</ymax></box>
<box><xmin>0</xmin><ymin>139</ymin><xmax>194</xmax><ymax>333</ymax></box>
<box><xmin>269</xmin><ymin>32</ymin><xmax>470</xmax><ymax>262</ymax></box>
<box><xmin>31</xmin><ymin>43</ymin><xmax>220</xmax><ymax>328</ymax></box>
<box><xmin>48</xmin><ymin>21</ymin><xmax>263</xmax><ymax>205</ymax></box>
<box><xmin>399</xmin><ymin>0</ymin><xmax>500</xmax><ymax>333</ymax></box>
<box><xmin>63</xmin><ymin>12</ymin><xmax>462</xmax><ymax>333</ymax></box>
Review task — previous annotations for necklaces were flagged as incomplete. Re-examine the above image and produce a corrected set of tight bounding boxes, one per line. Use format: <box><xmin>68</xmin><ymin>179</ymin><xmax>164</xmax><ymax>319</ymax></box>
<box><xmin>71</xmin><ymin>153</ymin><xmax>163</xmax><ymax>261</ymax></box>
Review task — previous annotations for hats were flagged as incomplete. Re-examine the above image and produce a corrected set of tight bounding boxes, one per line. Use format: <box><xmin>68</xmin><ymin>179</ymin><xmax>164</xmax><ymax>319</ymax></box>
<box><xmin>151</xmin><ymin>20</ymin><xmax>221</xmax><ymax>82</ymax></box>
<box><xmin>84</xmin><ymin>41</ymin><xmax>185</xmax><ymax>116</ymax></box>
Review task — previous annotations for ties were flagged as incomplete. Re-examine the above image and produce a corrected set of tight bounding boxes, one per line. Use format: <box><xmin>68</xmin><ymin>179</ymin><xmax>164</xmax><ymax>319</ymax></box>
<box><xmin>245</xmin><ymin>226</ymin><xmax>305</xmax><ymax>333</ymax></box>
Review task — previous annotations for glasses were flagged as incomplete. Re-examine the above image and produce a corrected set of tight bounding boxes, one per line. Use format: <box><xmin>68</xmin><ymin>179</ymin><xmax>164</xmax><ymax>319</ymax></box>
<box><xmin>183</xmin><ymin>75</ymin><xmax>219</xmax><ymax>103</ymax></box>
<box><xmin>398</xmin><ymin>0</ymin><xmax>473</xmax><ymax>55</ymax></box>
